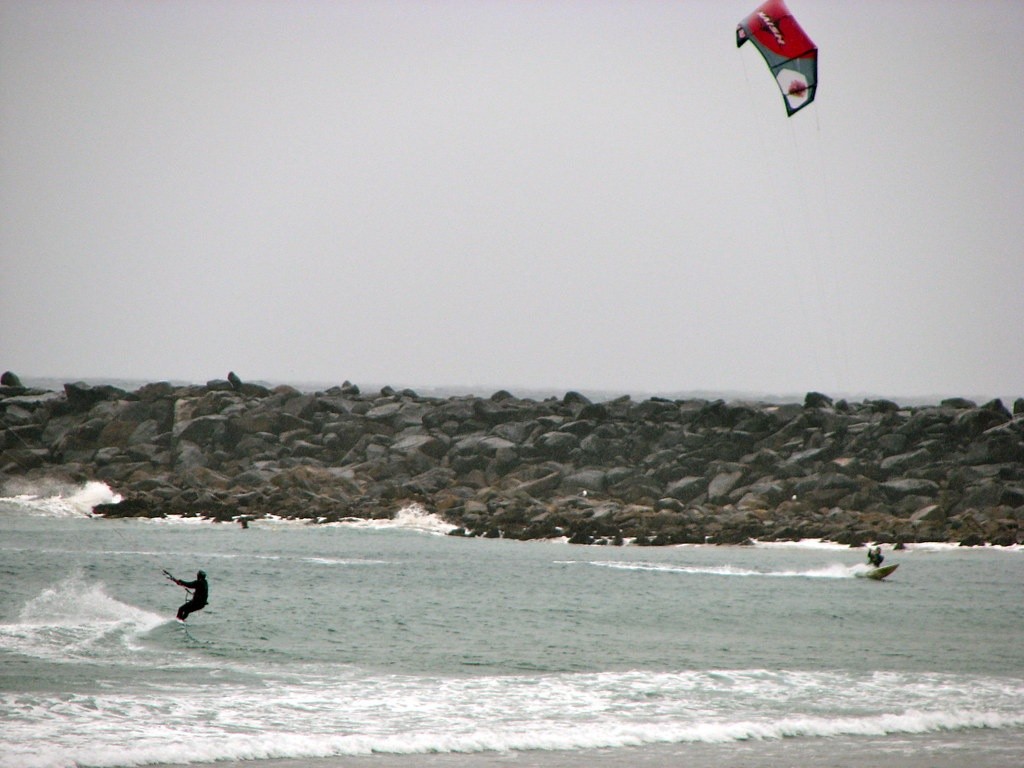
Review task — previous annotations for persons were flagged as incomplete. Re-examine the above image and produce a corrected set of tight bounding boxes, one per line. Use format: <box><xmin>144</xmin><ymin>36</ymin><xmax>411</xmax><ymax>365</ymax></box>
<box><xmin>867</xmin><ymin>546</ymin><xmax>883</xmax><ymax>568</ymax></box>
<box><xmin>176</xmin><ymin>570</ymin><xmax>209</xmax><ymax>622</ymax></box>
